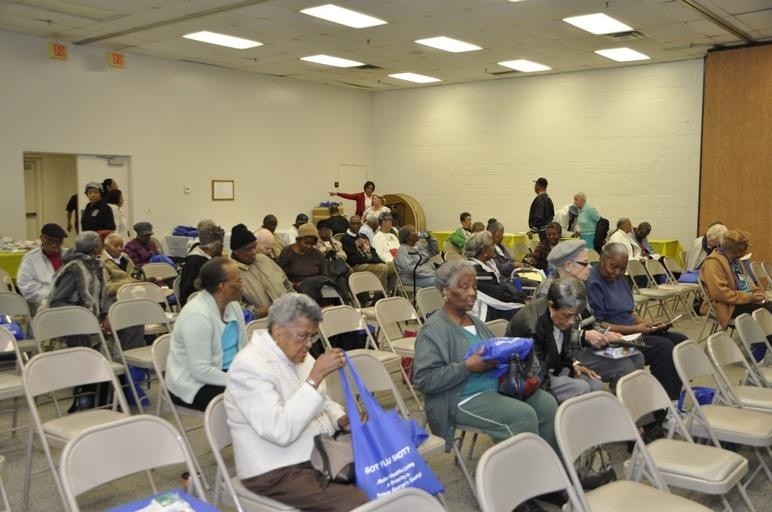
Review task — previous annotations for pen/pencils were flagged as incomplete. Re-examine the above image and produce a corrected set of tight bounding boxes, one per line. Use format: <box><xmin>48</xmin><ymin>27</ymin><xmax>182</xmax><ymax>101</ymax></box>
<box><xmin>604</xmin><ymin>326</ymin><xmax>612</xmax><ymax>336</ymax></box>
<box><xmin>670</xmin><ymin>314</ymin><xmax>682</xmax><ymax>323</ymax></box>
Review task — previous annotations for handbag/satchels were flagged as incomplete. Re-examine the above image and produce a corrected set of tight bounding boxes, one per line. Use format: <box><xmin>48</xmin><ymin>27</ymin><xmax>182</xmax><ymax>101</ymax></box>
<box><xmin>309</xmin><ymin>427</ymin><xmax>357</xmax><ymax>495</ymax></box>
<box><xmin>462</xmin><ymin>335</ymin><xmax>534</xmax><ymax>380</ymax></box>
<box><xmin>332</xmin><ymin>345</ymin><xmax>446</xmax><ymax>503</ymax></box>
<box><xmin>120</xmin><ymin>364</ymin><xmax>153</xmax><ymax>407</ymax></box>
<box><xmin>497</xmin><ymin>343</ymin><xmax>548</xmax><ymax>403</ymax></box>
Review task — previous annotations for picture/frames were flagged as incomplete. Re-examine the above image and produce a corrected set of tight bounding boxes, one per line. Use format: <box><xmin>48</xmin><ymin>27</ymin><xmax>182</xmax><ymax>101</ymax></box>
<box><xmin>212</xmin><ymin>180</ymin><xmax>235</xmax><ymax>200</ymax></box>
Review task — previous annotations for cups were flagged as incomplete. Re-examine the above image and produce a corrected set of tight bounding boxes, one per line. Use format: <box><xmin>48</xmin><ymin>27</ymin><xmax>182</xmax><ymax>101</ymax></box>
<box><xmin>181</xmin><ymin>470</ymin><xmax>201</xmax><ymax>497</ymax></box>
<box><xmin>161</xmin><ymin>286</ymin><xmax>169</xmax><ymax>295</ymax></box>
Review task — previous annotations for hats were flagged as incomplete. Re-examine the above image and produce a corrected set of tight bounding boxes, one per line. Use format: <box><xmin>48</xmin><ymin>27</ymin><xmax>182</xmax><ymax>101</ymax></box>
<box><xmin>533</xmin><ymin>177</ymin><xmax>548</xmax><ymax>188</ymax></box>
<box><xmin>294</xmin><ymin>223</ymin><xmax>319</xmax><ymax>246</ymax></box>
<box><xmin>199</xmin><ymin>225</ymin><xmax>225</xmax><ymax>245</ymax></box>
<box><xmin>84</xmin><ymin>180</ymin><xmax>105</xmax><ymax>195</ymax></box>
<box><xmin>722</xmin><ymin>227</ymin><xmax>752</xmax><ymax>245</ymax></box>
<box><xmin>41</xmin><ymin>223</ymin><xmax>68</xmax><ymax>238</ymax></box>
<box><xmin>229</xmin><ymin>222</ymin><xmax>257</xmax><ymax>251</ymax></box>
<box><xmin>134</xmin><ymin>221</ymin><xmax>154</xmax><ymax>236</ymax></box>
<box><xmin>546</xmin><ymin>239</ymin><xmax>588</xmax><ymax>267</ymax></box>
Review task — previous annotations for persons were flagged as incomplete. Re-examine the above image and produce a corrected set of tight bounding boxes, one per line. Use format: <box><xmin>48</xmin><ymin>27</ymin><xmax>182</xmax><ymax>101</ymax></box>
<box><xmin>224</xmin><ymin>292</ymin><xmax>369</xmax><ymax>512</ymax></box>
<box><xmin>16</xmin><ymin>178</ymin><xmax>440</xmax><ymax>413</ymax></box>
<box><xmin>685</xmin><ymin>223</ymin><xmax>771</xmax><ymax>363</ymax></box>
<box><xmin>412</xmin><ymin>178</ymin><xmax>688</xmax><ymax>512</ymax></box>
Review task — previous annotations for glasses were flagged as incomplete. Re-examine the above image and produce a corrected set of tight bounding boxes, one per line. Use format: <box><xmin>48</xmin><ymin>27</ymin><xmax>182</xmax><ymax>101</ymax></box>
<box><xmin>284</xmin><ymin>327</ymin><xmax>321</xmax><ymax>345</ymax></box>
<box><xmin>571</xmin><ymin>259</ymin><xmax>588</xmax><ymax>267</ymax></box>
